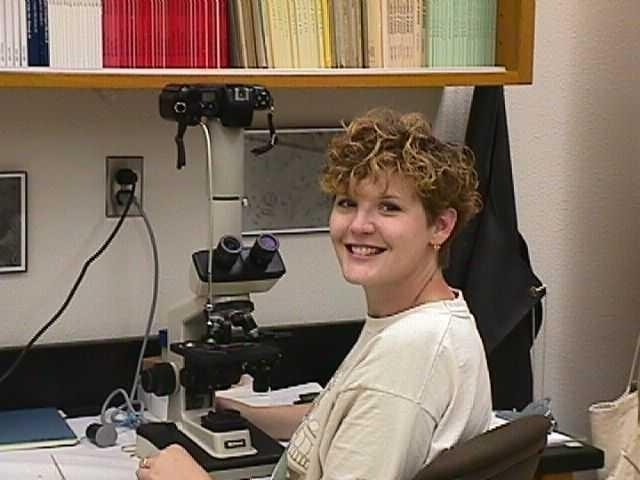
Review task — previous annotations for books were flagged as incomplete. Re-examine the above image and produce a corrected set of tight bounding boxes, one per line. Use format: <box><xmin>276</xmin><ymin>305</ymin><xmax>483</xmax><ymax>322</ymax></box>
<box><xmin>1</xmin><ymin>406</ymin><xmax>79</xmax><ymax>452</ymax></box>
<box><xmin>1</xmin><ymin>0</ymin><xmax>500</xmax><ymax>68</ymax></box>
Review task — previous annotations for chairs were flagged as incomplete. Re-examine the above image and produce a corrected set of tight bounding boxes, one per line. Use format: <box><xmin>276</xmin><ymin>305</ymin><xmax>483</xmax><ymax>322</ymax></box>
<box><xmin>409</xmin><ymin>412</ymin><xmax>554</xmax><ymax>479</ymax></box>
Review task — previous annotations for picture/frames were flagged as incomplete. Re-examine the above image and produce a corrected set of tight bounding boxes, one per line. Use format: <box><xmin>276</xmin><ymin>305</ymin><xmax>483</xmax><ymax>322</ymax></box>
<box><xmin>0</xmin><ymin>170</ymin><xmax>29</xmax><ymax>274</ymax></box>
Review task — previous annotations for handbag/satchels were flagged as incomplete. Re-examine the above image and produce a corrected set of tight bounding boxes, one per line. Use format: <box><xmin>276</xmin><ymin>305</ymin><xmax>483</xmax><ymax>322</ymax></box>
<box><xmin>587</xmin><ymin>380</ymin><xmax>640</xmax><ymax>480</ymax></box>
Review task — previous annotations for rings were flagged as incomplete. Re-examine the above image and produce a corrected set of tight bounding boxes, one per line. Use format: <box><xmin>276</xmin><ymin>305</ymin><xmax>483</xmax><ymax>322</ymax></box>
<box><xmin>141</xmin><ymin>457</ymin><xmax>147</xmax><ymax>467</ymax></box>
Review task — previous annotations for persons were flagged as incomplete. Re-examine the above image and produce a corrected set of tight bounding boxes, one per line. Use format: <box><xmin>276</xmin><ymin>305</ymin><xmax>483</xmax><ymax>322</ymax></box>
<box><xmin>134</xmin><ymin>108</ymin><xmax>496</xmax><ymax>479</ymax></box>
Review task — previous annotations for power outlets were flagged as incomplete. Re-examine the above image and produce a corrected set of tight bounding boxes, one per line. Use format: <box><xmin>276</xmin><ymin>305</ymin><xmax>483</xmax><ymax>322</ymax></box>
<box><xmin>103</xmin><ymin>154</ymin><xmax>144</xmax><ymax>219</ymax></box>
